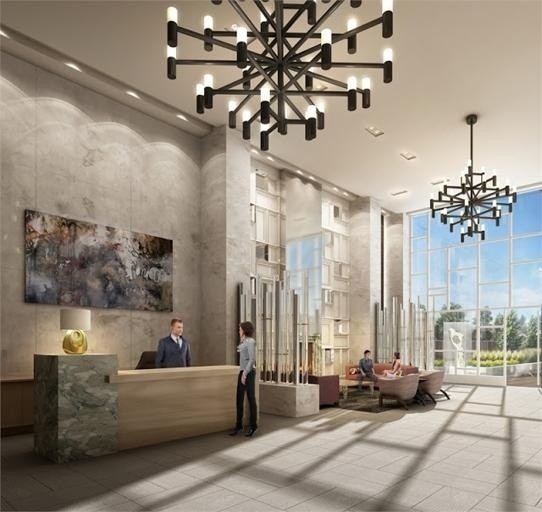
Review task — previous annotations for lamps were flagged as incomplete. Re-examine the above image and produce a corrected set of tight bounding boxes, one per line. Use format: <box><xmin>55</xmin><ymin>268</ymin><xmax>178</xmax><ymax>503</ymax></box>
<box><xmin>59</xmin><ymin>307</ymin><xmax>92</xmax><ymax>354</ymax></box>
<box><xmin>165</xmin><ymin>0</ymin><xmax>398</xmax><ymax>151</ymax></box>
<box><xmin>430</xmin><ymin>111</ymin><xmax>518</xmax><ymax>242</ymax></box>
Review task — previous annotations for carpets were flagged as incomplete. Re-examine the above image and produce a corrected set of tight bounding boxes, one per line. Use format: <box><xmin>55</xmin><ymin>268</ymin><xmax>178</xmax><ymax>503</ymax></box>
<box><xmin>338</xmin><ymin>391</ymin><xmax>450</xmax><ymax>413</ymax></box>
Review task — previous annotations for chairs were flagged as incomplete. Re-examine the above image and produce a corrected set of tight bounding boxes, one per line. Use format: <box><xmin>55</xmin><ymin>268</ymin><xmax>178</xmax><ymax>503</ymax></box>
<box><xmin>376</xmin><ymin>370</ymin><xmax>450</xmax><ymax>409</ymax></box>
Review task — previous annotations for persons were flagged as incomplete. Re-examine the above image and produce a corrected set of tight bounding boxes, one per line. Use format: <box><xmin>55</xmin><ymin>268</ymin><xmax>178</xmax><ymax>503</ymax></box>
<box><xmin>383</xmin><ymin>352</ymin><xmax>401</xmax><ymax>377</ymax></box>
<box><xmin>155</xmin><ymin>319</ymin><xmax>192</xmax><ymax>367</ymax></box>
<box><xmin>358</xmin><ymin>349</ymin><xmax>378</xmax><ymax>392</ymax></box>
<box><xmin>229</xmin><ymin>321</ymin><xmax>259</xmax><ymax>438</ymax></box>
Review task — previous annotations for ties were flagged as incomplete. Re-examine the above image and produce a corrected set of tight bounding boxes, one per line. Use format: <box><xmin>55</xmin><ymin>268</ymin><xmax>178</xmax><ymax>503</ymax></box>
<box><xmin>176</xmin><ymin>338</ymin><xmax>180</xmax><ymax>350</ymax></box>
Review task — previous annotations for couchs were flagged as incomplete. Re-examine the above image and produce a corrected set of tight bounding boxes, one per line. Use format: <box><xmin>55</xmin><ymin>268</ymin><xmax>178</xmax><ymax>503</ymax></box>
<box><xmin>261</xmin><ymin>371</ymin><xmax>339</xmax><ymax>409</ymax></box>
<box><xmin>345</xmin><ymin>363</ymin><xmax>418</xmax><ymax>389</ymax></box>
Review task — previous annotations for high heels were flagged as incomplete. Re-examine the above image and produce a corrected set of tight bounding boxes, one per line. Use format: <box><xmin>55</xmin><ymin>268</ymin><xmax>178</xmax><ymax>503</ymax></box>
<box><xmin>229</xmin><ymin>424</ymin><xmax>259</xmax><ymax>438</ymax></box>
